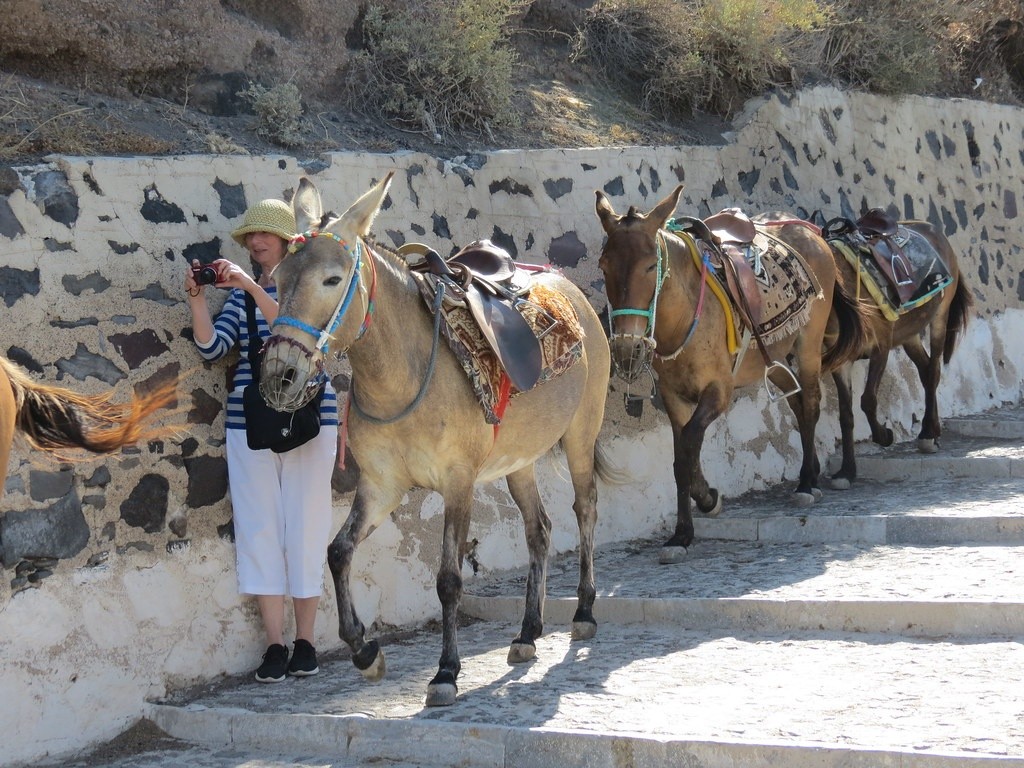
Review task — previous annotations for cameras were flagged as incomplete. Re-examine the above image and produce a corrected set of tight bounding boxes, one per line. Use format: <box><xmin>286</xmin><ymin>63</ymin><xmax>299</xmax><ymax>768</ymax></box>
<box><xmin>191</xmin><ymin>262</ymin><xmax>226</xmax><ymax>286</ymax></box>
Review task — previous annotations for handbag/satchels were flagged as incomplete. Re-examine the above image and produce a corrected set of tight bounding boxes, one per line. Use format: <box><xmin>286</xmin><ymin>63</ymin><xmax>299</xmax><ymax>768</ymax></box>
<box><xmin>244</xmin><ymin>379</ymin><xmax>326</xmax><ymax>452</ymax></box>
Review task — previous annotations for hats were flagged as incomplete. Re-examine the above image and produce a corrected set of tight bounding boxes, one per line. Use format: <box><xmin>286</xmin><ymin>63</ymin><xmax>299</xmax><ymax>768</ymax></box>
<box><xmin>232</xmin><ymin>199</ymin><xmax>296</xmax><ymax>251</ymax></box>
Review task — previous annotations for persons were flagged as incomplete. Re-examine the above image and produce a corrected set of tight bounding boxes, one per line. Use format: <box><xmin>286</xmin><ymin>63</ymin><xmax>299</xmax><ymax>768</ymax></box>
<box><xmin>185</xmin><ymin>198</ymin><xmax>339</xmax><ymax>682</ymax></box>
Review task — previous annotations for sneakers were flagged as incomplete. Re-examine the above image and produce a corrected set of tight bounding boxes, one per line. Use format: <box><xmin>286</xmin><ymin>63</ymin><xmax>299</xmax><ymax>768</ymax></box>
<box><xmin>255</xmin><ymin>644</ymin><xmax>289</xmax><ymax>682</ymax></box>
<box><xmin>289</xmin><ymin>640</ymin><xmax>319</xmax><ymax>676</ymax></box>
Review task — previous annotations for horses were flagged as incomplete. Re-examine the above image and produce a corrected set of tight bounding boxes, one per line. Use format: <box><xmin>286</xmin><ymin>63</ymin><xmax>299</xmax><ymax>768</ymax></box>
<box><xmin>594</xmin><ymin>185</ymin><xmax>884</xmax><ymax>565</ymax></box>
<box><xmin>258</xmin><ymin>169</ymin><xmax>628</xmax><ymax>707</ymax></box>
<box><xmin>0</xmin><ymin>355</ymin><xmax>202</xmax><ymax>515</ymax></box>
<box><xmin>796</xmin><ymin>203</ymin><xmax>974</xmax><ymax>490</ymax></box>
